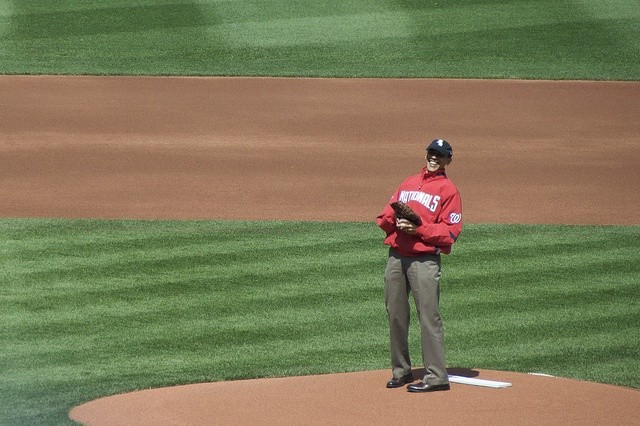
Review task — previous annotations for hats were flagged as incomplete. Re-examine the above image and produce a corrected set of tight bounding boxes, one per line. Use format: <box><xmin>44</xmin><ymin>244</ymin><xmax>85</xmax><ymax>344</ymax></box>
<box><xmin>425</xmin><ymin>139</ymin><xmax>453</xmax><ymax>156</ymax></box>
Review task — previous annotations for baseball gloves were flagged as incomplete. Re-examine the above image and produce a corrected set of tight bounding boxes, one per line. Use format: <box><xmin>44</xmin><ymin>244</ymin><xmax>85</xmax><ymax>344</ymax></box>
<box><xmin>390</xmin><ymin>199</ymin><xmax>422</xmax><ymax>227</ymax></box>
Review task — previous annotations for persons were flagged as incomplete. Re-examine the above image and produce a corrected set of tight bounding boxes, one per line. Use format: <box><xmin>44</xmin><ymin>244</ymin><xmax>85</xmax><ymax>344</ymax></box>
<box><xmin>375</xmin><ymin>138</ymin><xmax>463</xmax><ymax>392</ymax></box>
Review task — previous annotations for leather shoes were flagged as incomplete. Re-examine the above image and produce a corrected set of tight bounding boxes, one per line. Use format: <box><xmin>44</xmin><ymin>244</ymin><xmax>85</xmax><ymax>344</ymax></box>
<box><xmin>407</xmin><ymin>381</ymin><xmax>450</xmax><ymax>393</ymax></box>
<box><xmin>386</xmin><ymin>372</ymin><xmax>415</xmax><ymax>388</ymax></box>
